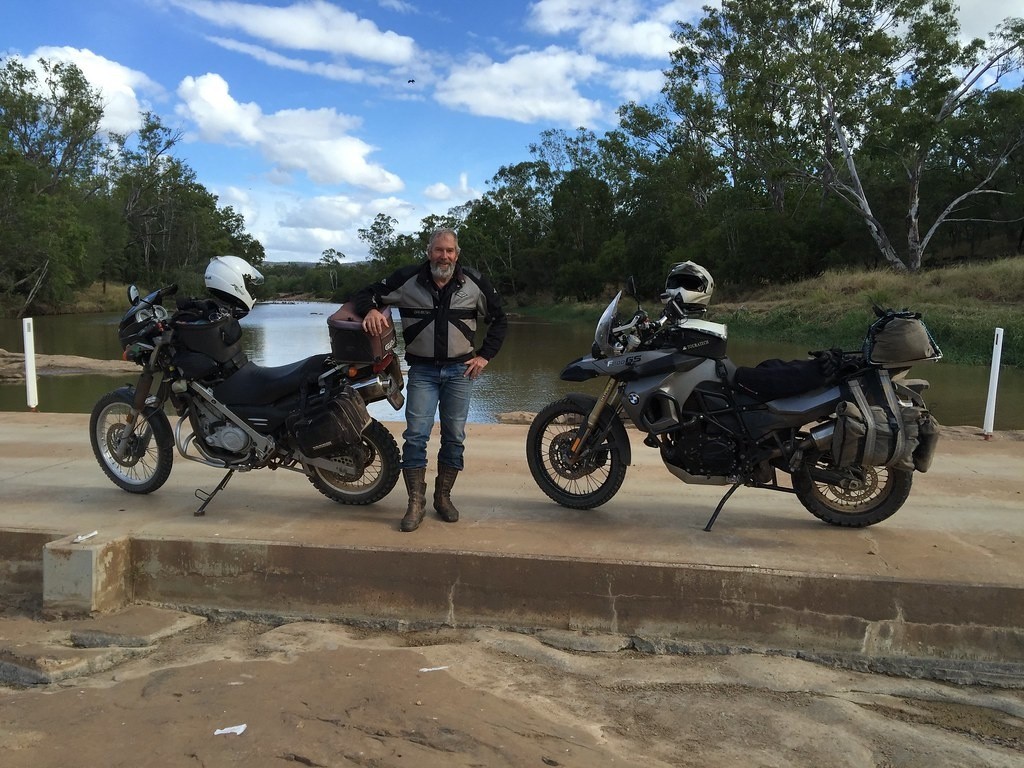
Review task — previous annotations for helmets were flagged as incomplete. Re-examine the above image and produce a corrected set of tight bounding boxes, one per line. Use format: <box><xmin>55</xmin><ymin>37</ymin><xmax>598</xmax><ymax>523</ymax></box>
<box><xmin>665</xmin><ymin>260</ymin><xmax>714</xmax><ymax>309</ymax></box>
<box><xmin>204</xmin><ymin>256</ymin><xmax>264</xmax><ymax>312</ymax></box>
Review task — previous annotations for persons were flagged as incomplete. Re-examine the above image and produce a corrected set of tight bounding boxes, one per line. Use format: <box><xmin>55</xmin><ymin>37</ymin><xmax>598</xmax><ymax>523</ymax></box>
<box><xmin>352</xmin><ymin>229</ymin><xmax>508</xmax><ymax>534</ymax></box>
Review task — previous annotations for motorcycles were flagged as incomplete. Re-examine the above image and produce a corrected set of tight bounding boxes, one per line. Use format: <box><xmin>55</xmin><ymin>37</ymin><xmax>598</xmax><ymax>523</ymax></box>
<box><xmin>526</xmin><ymin>275</ymin><xmax>943</xmax><ymax>532</ymax></box>
<box><xmin>89</xmin><ymin>283</ymin><xmax>406</xmax><ymax>517</ymax></box>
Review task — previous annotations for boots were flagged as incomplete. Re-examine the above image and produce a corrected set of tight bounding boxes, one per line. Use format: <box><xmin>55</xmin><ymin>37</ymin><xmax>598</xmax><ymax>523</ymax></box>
<box><xmin>401</xmin><ymin>468</ymin><xmax>427</xmax><ymax>531</ymax></box>
<box><xmin>433</xmin><ymin>463</ymin><xmax>459</xmax><ymax>523</ymax></box>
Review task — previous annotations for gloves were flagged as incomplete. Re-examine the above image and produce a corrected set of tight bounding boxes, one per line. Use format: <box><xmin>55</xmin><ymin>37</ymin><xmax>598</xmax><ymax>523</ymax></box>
<box><xmin>808</xmin><ymin>349</ymin><xmax>844</xmax><ymax>377</ymax></box>
<box><xmin>172</xmin><ymin>296</ymin><xmax>221</xmax><ymax>322</ymax></box>
